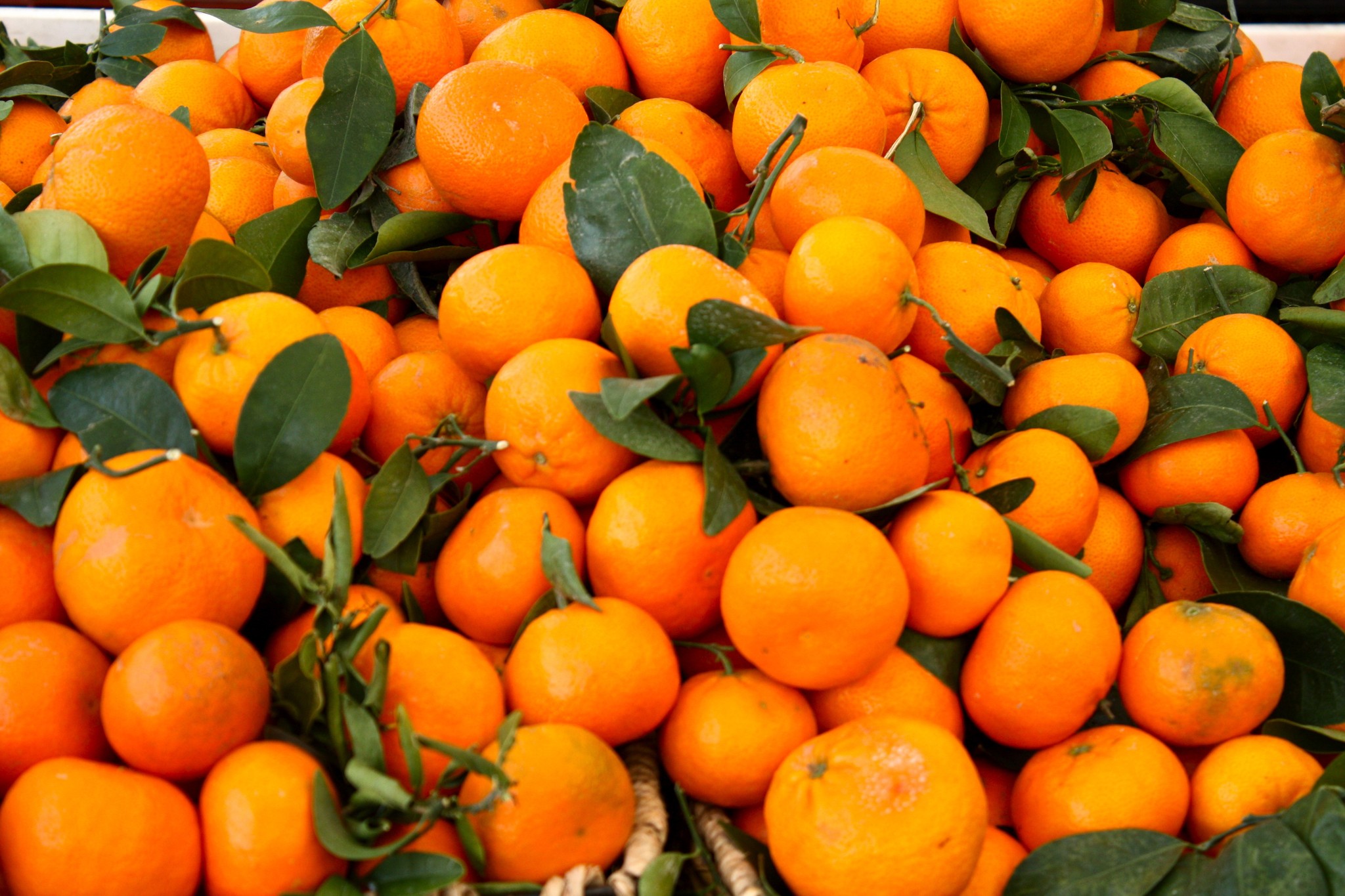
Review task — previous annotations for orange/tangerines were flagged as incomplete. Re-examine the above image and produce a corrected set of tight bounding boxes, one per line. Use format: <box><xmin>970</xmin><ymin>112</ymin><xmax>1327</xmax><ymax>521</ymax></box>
<box><xmin>0</xmin><ymin>0</ymin><xmax>1345</xmax><ymax>895</ymax></box>
